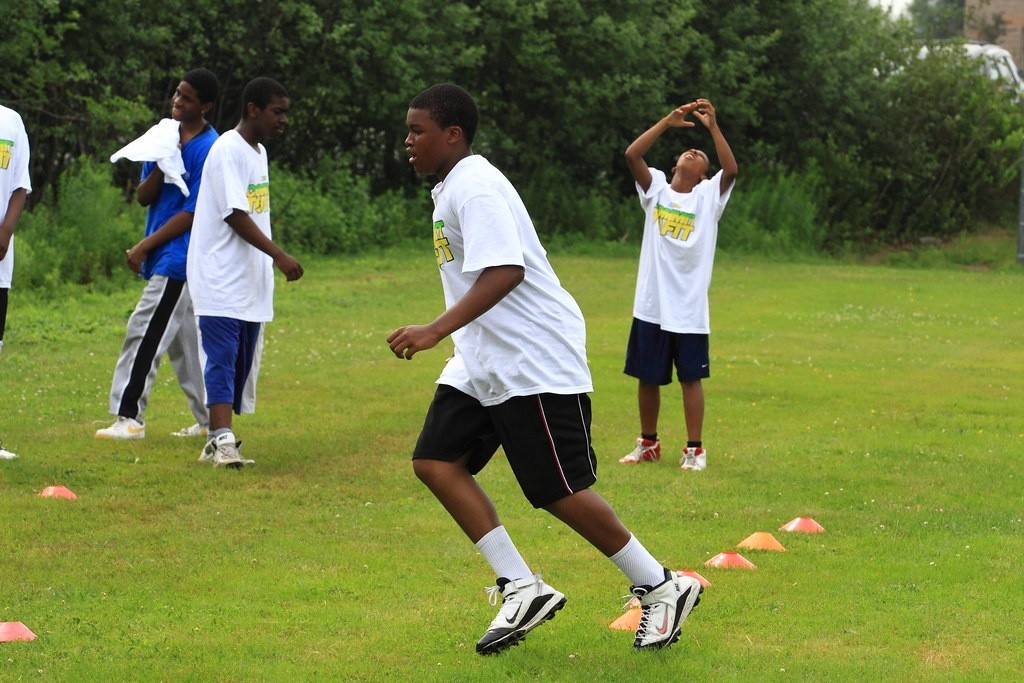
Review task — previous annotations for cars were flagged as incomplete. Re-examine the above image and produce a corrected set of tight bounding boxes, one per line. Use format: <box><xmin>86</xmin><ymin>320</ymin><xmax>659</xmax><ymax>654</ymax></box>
<box><xmin>870</xmin><ymin>44</ymin><xmax>1024</xmax><ymax>110</ymax></box>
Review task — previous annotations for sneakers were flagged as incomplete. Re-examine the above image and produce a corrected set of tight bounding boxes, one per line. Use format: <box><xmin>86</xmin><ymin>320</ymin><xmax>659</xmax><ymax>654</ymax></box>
<box><xmin>170</xmin><ymin>424</ymin><xmax>211</xmax><ymax>436</ymax></box>
<box><xmin>476</xmin><ymin>573</ymin><xmax>567</xmax><ymax>655</ymax></box>
<box><xmin>619</xmin><ymin>438</ymin><xmax>662</xmax><ymax>464</ymax></box>
<box><xmin>92</xmin><ymin>415</ymin><xmax>146</xmax><ymax>440</ymax></box>
<box><xmin>629</xmin><ymin>568</ymin><xmax>703</xmax><ymax>651</ymax></box>
<box><xmin>199</xmin><ymin>432</ymin><xmax>255</xmax><ymax>469</ymax></box>
<box><xmin>682</xmin><ymin>447</ymin><xmax>708</xmax><ymax>473</ymax></box>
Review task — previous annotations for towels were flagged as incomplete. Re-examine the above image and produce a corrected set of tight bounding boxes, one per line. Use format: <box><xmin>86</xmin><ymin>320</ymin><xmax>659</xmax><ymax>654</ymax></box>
<box><xmin>110</xmin><ymin>117</ymin><xmax>191</xmax><ymax>198</ymax></box>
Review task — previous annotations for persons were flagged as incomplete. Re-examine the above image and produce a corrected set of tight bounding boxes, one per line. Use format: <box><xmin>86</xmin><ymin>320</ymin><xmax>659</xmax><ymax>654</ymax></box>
<box><xmin>386</xmin><ymin>83</ymin><xmax>704</xmax><ymax>656</ymax></box>
<box><xmin>620</xmin><ymin>100</ymin><xmax>739</xmax><ymax>471</ymax></box>
<box><xmin>185</xmin><ymin>77</ymin><xmax>304</xmax><ymax>467</ymax></box>
<box><xmin>0</xmin><ymin>104</ymin><xmax>33</xmax><ymax>459</ymax></box>
<box><xmin>93</xmin><ymin>71</ymin><xmax>221</xmax><ymax>441</ymax></box>
